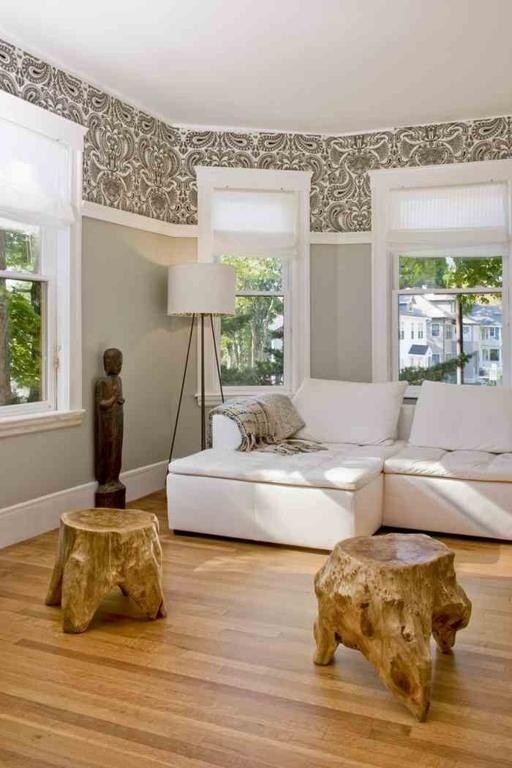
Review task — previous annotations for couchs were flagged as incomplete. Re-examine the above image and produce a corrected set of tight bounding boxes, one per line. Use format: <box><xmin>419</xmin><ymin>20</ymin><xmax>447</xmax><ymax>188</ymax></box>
<box><xmin>212</xmin><ymin>377</ymin><xmax>512</xmax><ymax>548</ymax></box>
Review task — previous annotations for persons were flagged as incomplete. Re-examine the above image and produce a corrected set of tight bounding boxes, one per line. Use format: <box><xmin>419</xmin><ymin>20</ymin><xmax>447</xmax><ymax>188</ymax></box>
<box><xmin>93</xmin><ymin>348</ymin><xmax>128</xmax><ymax>494</ymax></box>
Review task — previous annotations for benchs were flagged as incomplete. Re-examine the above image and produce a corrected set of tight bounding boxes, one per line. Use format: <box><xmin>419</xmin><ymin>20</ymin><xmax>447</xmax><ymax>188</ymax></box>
<box><xmin>165</xmin><ymin>445</ymin><xmax>386</xmax><ymax>553</ymax></box>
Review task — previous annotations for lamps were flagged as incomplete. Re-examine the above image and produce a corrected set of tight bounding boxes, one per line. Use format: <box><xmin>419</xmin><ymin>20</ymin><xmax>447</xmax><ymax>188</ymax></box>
<box><xmin>163</xmin><ymin>262</ymin><xmax>229</xmax><ymax>501</ymax></box>
